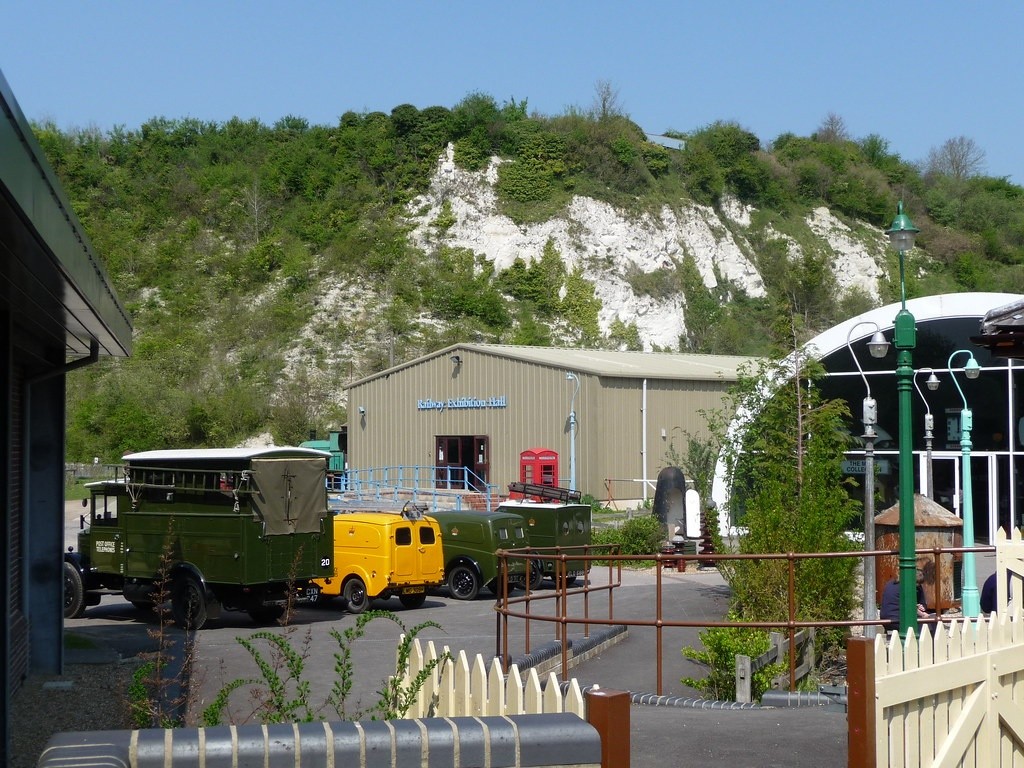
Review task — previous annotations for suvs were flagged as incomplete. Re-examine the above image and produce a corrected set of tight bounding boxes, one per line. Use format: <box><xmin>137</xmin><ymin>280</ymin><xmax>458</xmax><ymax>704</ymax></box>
<box><xmin>494</xmin><ymin>480</ymin><xmax>592</xmax><ymax>587</ymax></box>
<box><xmin>424</xmin><ymin>511</ymin><xmax>533</xmax><ymax>600</ymax></box>
<box><xmin>64</xmin><ymin>447</ymin><xmax>338</xmax><ymax>630</ymax></box>
<box><xmin>298</xmin><ymin>509</ymin><xmax>445</xmax><ymax>614</ymax></box>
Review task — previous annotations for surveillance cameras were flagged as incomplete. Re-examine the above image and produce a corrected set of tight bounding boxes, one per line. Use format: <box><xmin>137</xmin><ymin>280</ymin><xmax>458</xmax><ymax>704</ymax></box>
<box><xmin>359</xmin><ymin>407</ymin><xmax>365</xmax><ymax>414</ymax></box>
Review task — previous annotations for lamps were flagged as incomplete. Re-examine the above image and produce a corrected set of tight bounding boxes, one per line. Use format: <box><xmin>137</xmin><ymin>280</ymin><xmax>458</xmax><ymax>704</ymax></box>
<box><xmin>450</xmin><ymin>356</ymin><xmax>463</xmax><ymax>365</ymax></box>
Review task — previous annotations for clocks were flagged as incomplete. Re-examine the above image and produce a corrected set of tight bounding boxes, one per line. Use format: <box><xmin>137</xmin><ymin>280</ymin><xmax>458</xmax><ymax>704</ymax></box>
<box><xmin>947</xmin><ymin>414</ymin><xmax>962</xmax><ymax>441</ymax></box>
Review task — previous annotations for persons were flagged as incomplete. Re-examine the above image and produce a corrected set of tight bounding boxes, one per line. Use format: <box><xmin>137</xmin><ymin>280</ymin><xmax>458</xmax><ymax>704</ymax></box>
<box><xmin>979</xmin><ymin>569</ymin><xmax>1012</xmax><ymax>613</ymax></box>
<box><xmin>880</xmin><ymin>565</ymin><xmax>937</xmax><ymax>639</ymax></box>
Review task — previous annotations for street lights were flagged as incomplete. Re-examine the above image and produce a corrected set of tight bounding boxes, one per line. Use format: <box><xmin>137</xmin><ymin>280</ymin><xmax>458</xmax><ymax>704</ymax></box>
<box><xmin>913</xmin><ymin>365</ymin><xmax>941</xmax><ymax>498</ymax></box>
<box><xmin>946</xmin><ymin>349</ymin><xmax>983</xmax><ymax>620</ymax></box>
<box><xmin>846</xmin><ymin>319</ymin><xmax>894</xmax><ymax>642</ymax></box>
<box><xmin>884</xmin><ymin>199</ymin><xmax>922</xmax><ymax>640</ymax></box>
<box><xmin>566</xmin><ymin>371</ymin><xmax>581</xmax><ymax>494</ymax></box>
